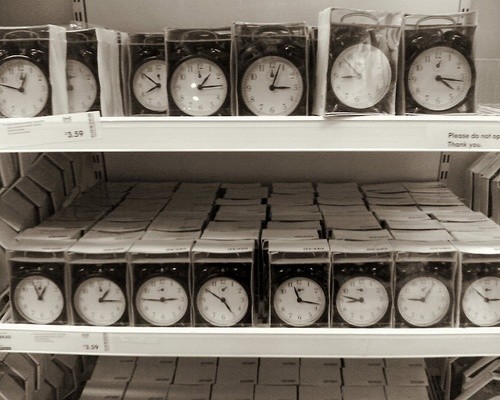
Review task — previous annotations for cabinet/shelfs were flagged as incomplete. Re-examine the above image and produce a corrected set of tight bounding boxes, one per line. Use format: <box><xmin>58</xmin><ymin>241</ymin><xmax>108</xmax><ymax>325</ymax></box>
<box><xmin>0</xmin><ymin>104</ymin><xmax>500</xmax><ymax>359</ymax></box>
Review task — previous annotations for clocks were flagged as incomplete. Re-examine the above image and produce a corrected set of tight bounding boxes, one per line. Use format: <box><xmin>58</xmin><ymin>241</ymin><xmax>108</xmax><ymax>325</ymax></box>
<box><xmin>0</xmin><ymin>30</ymin><xmax>51</xmax><ymax>118</ymax></box>
<box><xmin>404</xmin><ymin>14</ymin><xmax>477</xmax><ymax>113</ymax></box>
<box><xmin>328</xmin><ymin>11</ymin><xmax>397</xmax><ymax>114</ymax></box>
<box><xmin>72</xmin><ymin>253</ymin><xmax>127</xmax><ymax>326</ymax></box>
<box><xmin>67</xmin><ymin>32</ymin><xmax>100</xmax><ymax>113</ymax></box>
<box><xmin>271</xmin><ymin>252</ymin><xmax>329</xmax><ymax>328</ymax></box>
<box><xmin>167</xmin><ymin>29</ymin><xmax>230</xmax><ymax>116</ymax></box>
<box><xmin>130</xmin><ymin>36</ymin><xmax>167</xmax><ymax>114</ymax></box>
<box><xmin>333</xmin><ymin>253</ymin><xmax>392</xmax><ymax>328</ymax></box>
<box><xmin>460</xmin><ymin>253</ymin><xmax>500</xmax><ymax>327</ymax></box>
<box><xmin>237</xmin><ymin>24</ymin><xmax>307</xmax><ymax>116</ymax></box>
<box><xmin>133</xmin><ymin>253</ymin><xmax>190</xmax><ymax>327</ymax></box>
<box><xmin>394</xmin><ymin>252</ymin><xmax>453</xmax><ymax>328</ymax></box>
<box><xmin>193</xmin><ymin>252</ymin><xmax>252</xmax><ymax>327</ymax></box>
<box><xmin>11</xmin><ymin>251</ymin><xmax>66</xmax><ymax>324</ymax></box>
